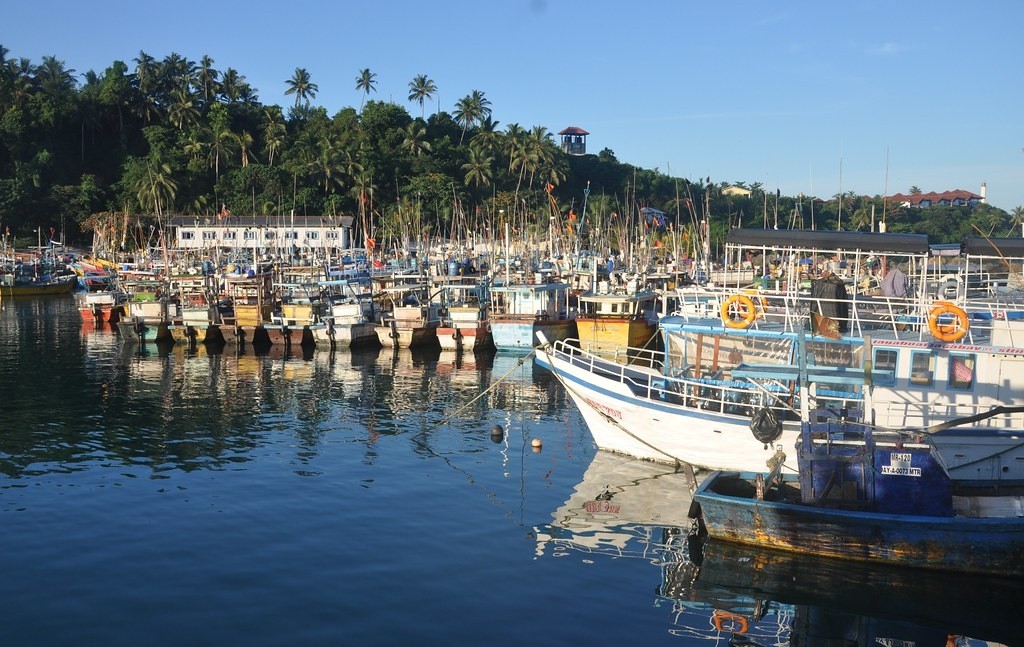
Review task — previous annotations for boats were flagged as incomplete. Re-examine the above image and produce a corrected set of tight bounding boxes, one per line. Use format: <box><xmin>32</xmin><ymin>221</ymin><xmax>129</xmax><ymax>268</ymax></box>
<box><xmin>533</xmin><ymin>153</ymin><xmax>1019</xmax><ymax>574</ymax></box>
<box><xmin>3</xmin><ymin>236</ymin><xmax>86</xmax><ymax>287</ymax></box>
<box><xmin>77</xmin><ymin>168</ymin><xmax>661</xmax><ymax>349</ymax></box>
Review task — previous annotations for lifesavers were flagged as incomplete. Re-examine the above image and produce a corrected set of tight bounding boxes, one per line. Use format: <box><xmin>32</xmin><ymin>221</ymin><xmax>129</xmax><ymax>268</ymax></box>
<box><xmin>939</xmin><ymin>273</ymin><xmax>964</xmax><ymax>285</ymax></box>
<box><xmin>930</xmin><ymin>304</ymin><xmax>969</xmax><ymax>342</ymax></box>
<box><xmin>738</xmin><ymin>290</ymin><xmax>767</xmax><ymax>319</ymax></box>
<box><xmin>929</xmin><ymin>300</ymin><xmax>958</xmax><ymax>332</ymax></box>
<box><xmin>937</xmin><ymin>281</ymin><xmax>964</xmax><ymax>306</ymax></box>
<box><xmin>720</xmin><ymin>295</ymin><xmax>755</xmax><ymax>328</ymax></box>
